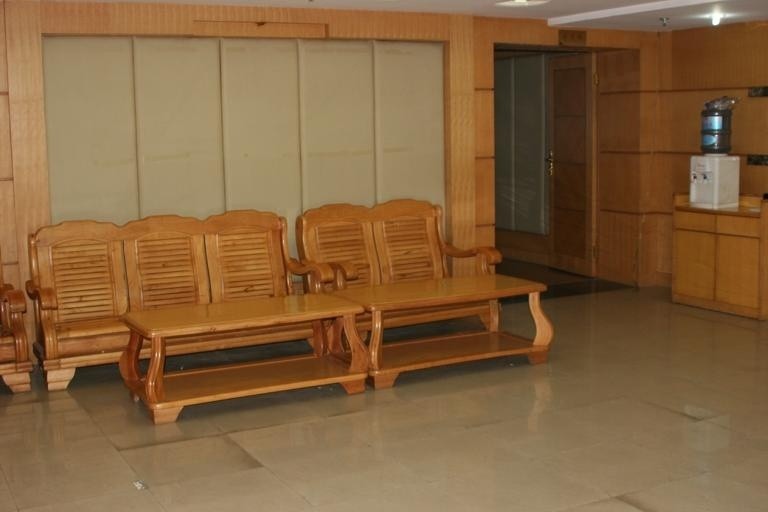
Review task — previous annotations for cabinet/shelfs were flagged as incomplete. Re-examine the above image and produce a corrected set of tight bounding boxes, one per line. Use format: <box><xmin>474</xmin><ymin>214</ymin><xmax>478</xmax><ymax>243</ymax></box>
<box><xmin>671</xmin><ymin>193</ymin><xmax>768</xmax><ymax>320</ymax></box>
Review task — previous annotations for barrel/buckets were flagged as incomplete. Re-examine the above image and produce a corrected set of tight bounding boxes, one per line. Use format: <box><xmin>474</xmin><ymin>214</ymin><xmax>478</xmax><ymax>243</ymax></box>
<box><xmin>700</xmin><ymin>107</ymin><xmax>732</xmax><ymax>154</ymax></box>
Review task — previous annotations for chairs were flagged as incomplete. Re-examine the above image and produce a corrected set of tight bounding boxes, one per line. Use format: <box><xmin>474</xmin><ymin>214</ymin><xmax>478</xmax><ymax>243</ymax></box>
<box><xmin>0</xmin><ymin>264</ymin><xmax>35</xmax><ymax>394</ymax></box>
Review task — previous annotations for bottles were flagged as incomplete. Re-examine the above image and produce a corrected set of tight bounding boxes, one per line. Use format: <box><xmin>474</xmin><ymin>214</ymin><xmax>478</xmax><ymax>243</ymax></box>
<box><xmin>704</xmin><ymin>95</ymin><xmax>742</xmax><ymax>109</ymax></box>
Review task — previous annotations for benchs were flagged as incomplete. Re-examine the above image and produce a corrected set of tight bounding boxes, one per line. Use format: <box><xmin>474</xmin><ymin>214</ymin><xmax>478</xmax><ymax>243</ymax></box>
<box><xmin>296</xmin><ymin>199</ymin><xmax>503</xmax><ymax>360</ymax></box>
<box><xmin>25</xmin><ymin>209</ymin><xmax>335</xmax><ymax>392</ymax></box>
